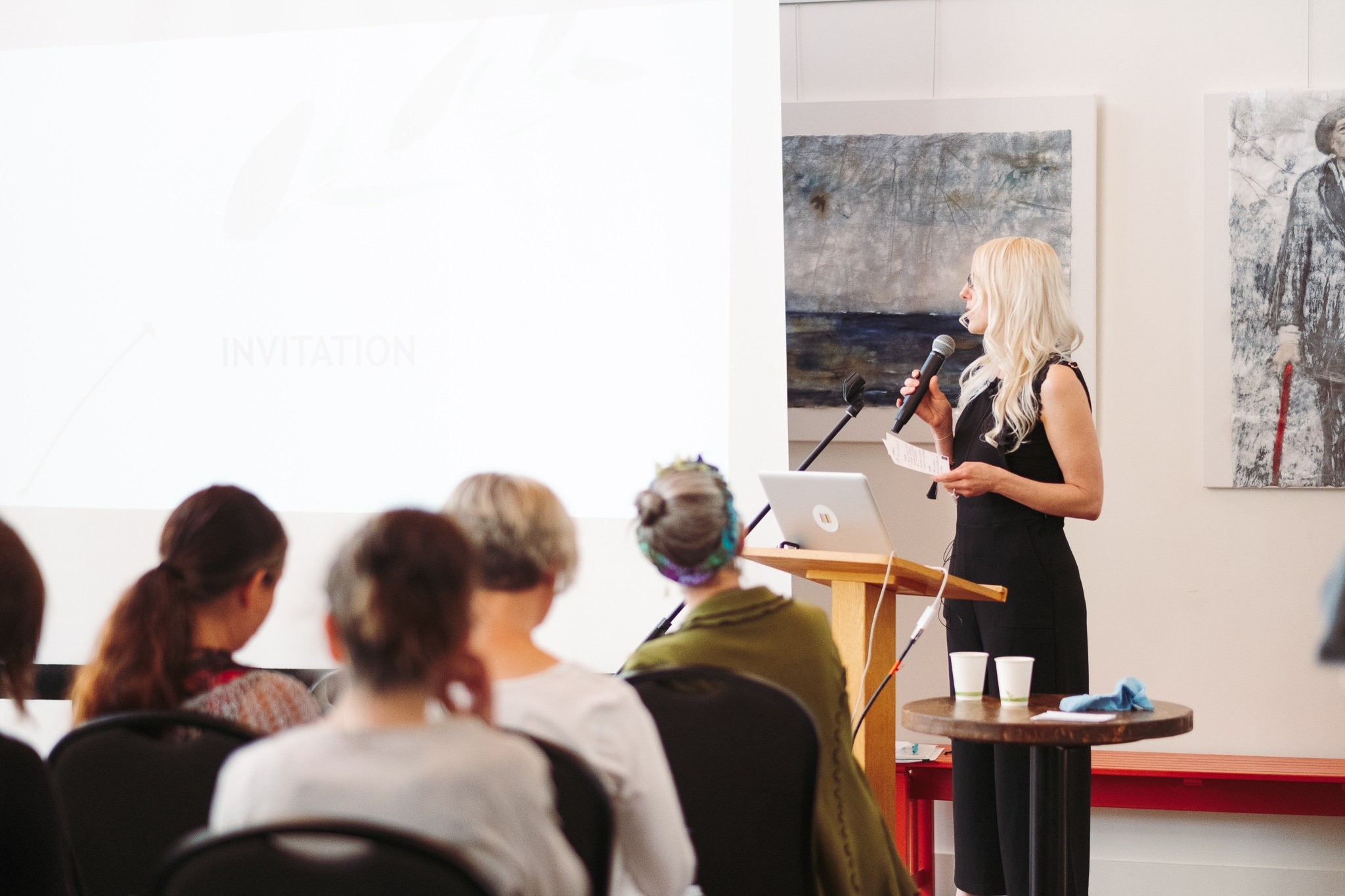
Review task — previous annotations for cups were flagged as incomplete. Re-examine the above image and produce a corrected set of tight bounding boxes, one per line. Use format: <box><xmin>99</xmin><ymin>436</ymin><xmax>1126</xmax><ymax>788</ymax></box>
<box><xmin>949</xmin><ymin>651</ymin><xmax>990</xmax><ymax>701</ymax></box>
<box><xmin>995</xmin><ymin>655</ymin><xmax>1035</xmax><ymax>706</ymax></box>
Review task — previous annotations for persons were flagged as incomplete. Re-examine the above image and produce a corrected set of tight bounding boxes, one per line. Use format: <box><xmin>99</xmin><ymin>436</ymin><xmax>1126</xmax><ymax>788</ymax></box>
<box><xmin>0</xmin><ymin>431</ymin><xmax>924</xmax><ymax>896</ymax></box>
<box><xmin>896</xmin><ymin>238</ymin><xmax>1105</xmax><ymax>896</ymax></box>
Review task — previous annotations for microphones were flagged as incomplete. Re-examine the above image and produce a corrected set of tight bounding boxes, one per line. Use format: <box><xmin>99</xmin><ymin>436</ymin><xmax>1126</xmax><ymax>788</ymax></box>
<box><xmin>892</xmin><ymin>334</ymin><xmax>955</xmax><ymax>433</ymax></box>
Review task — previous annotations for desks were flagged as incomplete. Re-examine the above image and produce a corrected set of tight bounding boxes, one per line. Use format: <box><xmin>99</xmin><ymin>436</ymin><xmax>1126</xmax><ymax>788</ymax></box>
<box><xmin>901</xmin><ymin>694</ymin><xmax>1192</xmax><ymax>896</ymax></box>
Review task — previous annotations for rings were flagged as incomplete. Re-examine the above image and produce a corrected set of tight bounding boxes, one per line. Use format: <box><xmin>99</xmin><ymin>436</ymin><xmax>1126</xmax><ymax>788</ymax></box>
<box><xmin>950</xmin><ymin>489</ymin><xmax>961</xmax><ymax>500</ymax></box>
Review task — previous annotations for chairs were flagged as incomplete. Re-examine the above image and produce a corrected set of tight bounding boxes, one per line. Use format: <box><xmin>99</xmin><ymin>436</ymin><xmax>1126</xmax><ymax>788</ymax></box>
<box><xmin>46</xmin><ymin>709</ymin><xmax>268</xmax><ymax>896</ymax></box>
<box><xmin>628</xmin><ymin>664</ymin><xmax>827</xmax><ymax>896</ymax></box>
<box><xmin>147</xmin><ymin>815</ymin><xmax>500</xmax><ymax>896</ymax></box>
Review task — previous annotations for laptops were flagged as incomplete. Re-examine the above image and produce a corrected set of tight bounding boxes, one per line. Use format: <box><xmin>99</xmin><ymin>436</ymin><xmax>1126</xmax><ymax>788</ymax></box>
<box><xmin>760</xmin><ymin>471</ymin><xmax>898</xmax><ymax>558</ymax></box>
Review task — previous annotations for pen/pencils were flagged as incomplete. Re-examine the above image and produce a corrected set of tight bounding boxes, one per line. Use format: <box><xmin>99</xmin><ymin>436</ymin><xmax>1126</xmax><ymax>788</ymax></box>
<box><xmin>912</xmin><ymin>742</ymin><xmax>918</xmax><ymax>753</ymax></box>
